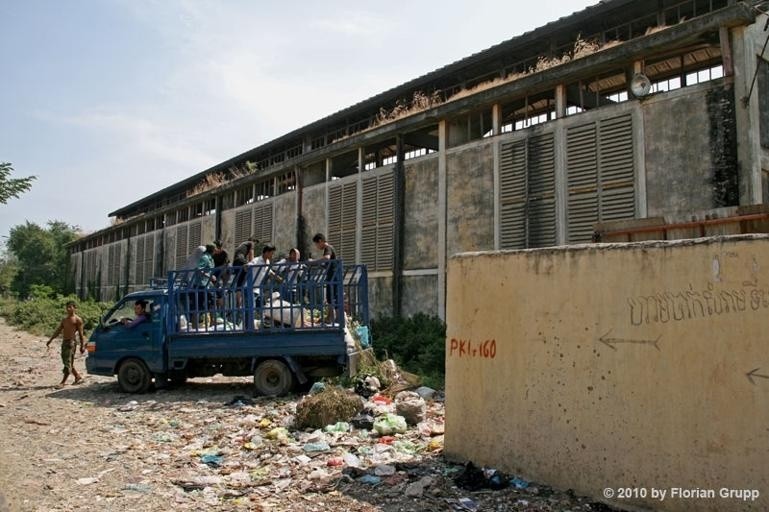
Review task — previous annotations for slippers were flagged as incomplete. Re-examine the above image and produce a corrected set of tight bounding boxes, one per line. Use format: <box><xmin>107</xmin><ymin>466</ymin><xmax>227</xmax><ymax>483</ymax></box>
<box><xmin>72</xmin><ymin>378</ymin><xmax>85</xmax><ymax>385</ymax></box>
<box><xmin>54</xmin><ymin>385</ymin><xmax>64</xmax><ymax>390</ymax></box>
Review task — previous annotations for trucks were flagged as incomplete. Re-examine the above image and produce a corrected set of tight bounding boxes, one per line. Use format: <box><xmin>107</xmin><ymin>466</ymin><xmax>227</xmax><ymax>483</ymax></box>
<box><xmin>85</xmin><ymin>259</ymin><xmax>372</xmax><ymax>397</ymax></box>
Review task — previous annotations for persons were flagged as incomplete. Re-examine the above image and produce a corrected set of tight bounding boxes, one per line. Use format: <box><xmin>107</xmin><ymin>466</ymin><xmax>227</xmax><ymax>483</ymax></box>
<box><xmin>121</xmin><ymin>301</ymin><xmax>152</xmax><ymax>328</ymax></box>
<box><xmin>178</xmin><ymin>233</ymin><xmax>338</xmax><ymax>327</ymax></box>
<box><xmin>47</xmin><ymin>301</ymin><xmax>84</xmax><ymax>389</ymax></box>
